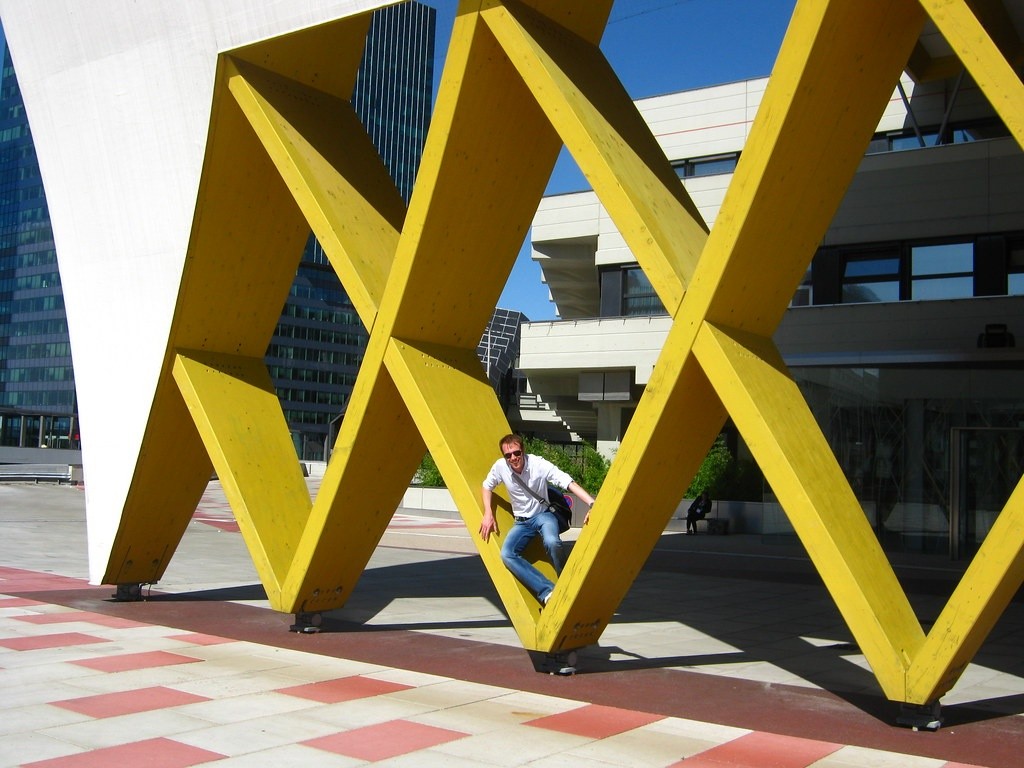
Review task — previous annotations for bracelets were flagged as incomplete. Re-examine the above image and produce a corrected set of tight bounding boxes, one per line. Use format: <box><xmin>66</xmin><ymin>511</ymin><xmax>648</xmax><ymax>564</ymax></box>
<box><xmin>589</xmin><ymin>502</ymin><xmax>594</xmax><ymax>509</ymax></box>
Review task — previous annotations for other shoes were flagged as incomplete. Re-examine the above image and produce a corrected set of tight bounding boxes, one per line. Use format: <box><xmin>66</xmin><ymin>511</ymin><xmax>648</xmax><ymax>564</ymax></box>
<box><xmin>543</xmin><ymin>589</ymin><xmax>553</xmax><ymax>604</ymax></box>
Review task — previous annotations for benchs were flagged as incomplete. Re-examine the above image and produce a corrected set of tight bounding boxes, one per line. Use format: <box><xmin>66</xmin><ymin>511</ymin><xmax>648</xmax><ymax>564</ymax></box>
<box><xmin>678</xmin><ymin>517</ymin><xmax>729</xmax><ymax>536</ymax></box>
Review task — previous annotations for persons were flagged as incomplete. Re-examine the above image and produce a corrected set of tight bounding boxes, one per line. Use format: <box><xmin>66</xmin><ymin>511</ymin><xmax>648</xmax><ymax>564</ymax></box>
<box><xmin>479</xmin><ymin>434</ymin><xmax>596</xmax><ymax>609</ymax></box>
<box><xmin>686</xmin><ymin>490</ymin><xmax>712</xmax><ymax>536</ymax></box>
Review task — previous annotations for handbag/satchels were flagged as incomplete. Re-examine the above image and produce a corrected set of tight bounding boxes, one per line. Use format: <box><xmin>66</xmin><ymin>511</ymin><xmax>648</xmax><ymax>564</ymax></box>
<box><xmin>546</xmin><ymin>484</ymin><xmax>572</xmax><ymax>534</ymax></box>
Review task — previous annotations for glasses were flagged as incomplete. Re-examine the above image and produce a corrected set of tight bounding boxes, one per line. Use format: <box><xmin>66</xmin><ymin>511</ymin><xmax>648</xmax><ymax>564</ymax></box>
<box><xmin>504</xmin><ymin>450</ymin><xmax>523</xmax><ymax>460</ymax></box>
